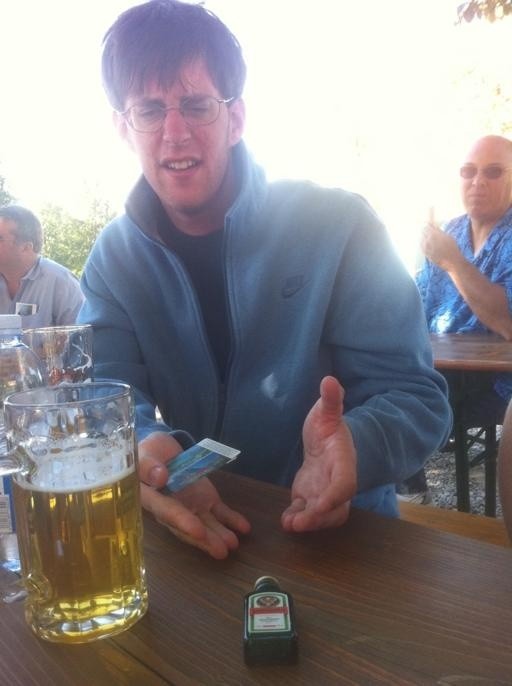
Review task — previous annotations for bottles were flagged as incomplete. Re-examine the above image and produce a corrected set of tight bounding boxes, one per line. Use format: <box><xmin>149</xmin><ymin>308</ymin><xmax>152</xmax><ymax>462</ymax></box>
<box><xmin>243</xmin><ymin>574</ymin><xmax>298</xmax><ymax>664</ymax></box>
<box><xmin>0</xmin><ymin>407</ymin><xmax>23</xmax><ymax>572</ymax></box>
<box><xmin>0</xmin><ymin>314</ymin><xmax>44</xmax><ymax>403</ymax></box>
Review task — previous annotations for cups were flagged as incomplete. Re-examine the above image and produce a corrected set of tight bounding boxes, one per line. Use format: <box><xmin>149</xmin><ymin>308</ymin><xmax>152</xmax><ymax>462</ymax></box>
<box><xmin>23</xmin><ymin>323</ymin><xmax>95</xmax><ymax>387</ymax></box>
<box><xmin>2</xmin><ymin>382</ymin><xmax>148</xmax><ymax>643</ymax></box>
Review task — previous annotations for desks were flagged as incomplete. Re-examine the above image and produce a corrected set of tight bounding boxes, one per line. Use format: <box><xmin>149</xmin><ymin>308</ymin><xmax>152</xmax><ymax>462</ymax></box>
<box><xmin>424</xmin><ymin>334</ymin><xmax>512</xmax><ymax>517</ymax></box>
<box><xmin>0</xmin><ymin>468</ymin><xmax>512</xmax><ymax>686</ymax></box>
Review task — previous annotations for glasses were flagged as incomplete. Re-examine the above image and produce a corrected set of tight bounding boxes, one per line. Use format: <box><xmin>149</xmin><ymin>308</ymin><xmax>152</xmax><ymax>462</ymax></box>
<box><xmin>459</xmin><ymin>166</ymin><xmax>512</xmax><ymax>179</ymax></box>
<box><xmin>114</xmin><ymin>96</ymin><xmax>235</xmax><ymax>133</ymax></box>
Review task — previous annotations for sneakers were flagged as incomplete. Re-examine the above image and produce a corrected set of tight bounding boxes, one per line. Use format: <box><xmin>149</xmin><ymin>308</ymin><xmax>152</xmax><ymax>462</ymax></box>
<box><xmin>396</xmin><ymin>488</ymin><xmax>433</xmax><ymax>506</ymax></box>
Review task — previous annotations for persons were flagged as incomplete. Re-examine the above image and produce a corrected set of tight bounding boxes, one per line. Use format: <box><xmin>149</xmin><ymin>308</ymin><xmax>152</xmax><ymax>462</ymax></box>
<box><xmin>62</xmin><ymin>0</ymin><xmax>455</xmax><ymax>561</ymax></box>
<box><xmin>0</xmin><ymin>205</ymin><xmax>85</xmax><ymax>330</ymax></box>
<box><xmin>395</xmin><ymin>134</ymin><xmax>511</xmax><ymax>544</ymax></box>
